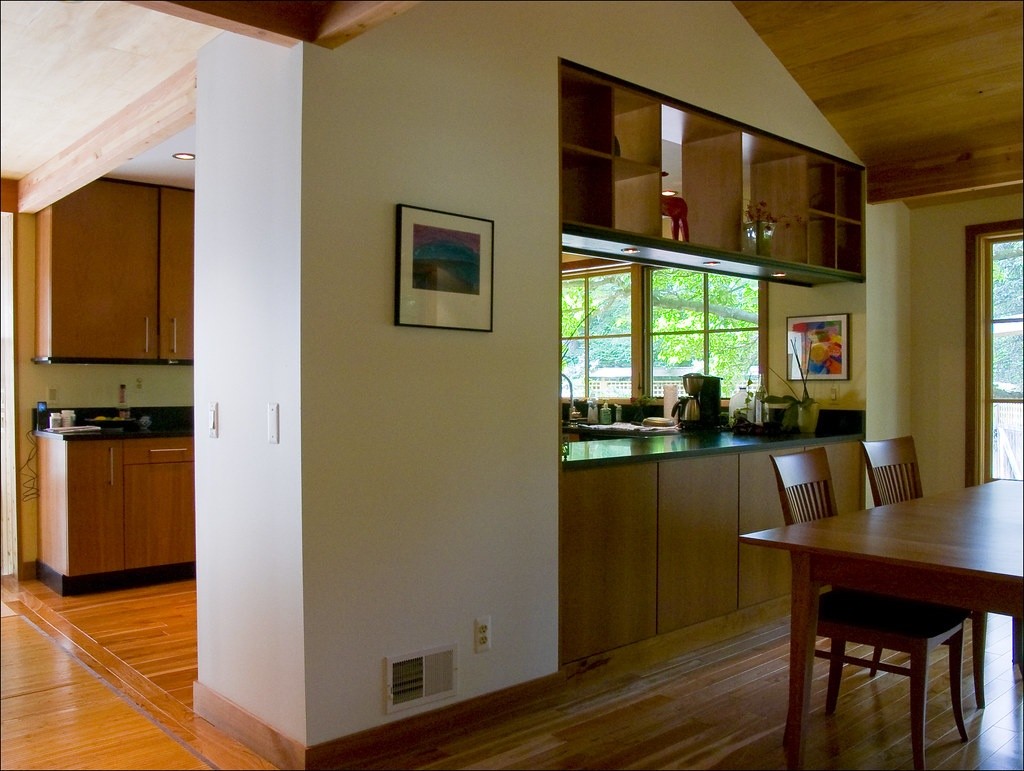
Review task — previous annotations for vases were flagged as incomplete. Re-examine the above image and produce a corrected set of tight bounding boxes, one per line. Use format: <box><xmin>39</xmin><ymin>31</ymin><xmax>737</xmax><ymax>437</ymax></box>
<box><xmin>745</xmin><ymin>221</ymin><xmax>776</xmax><ymax>258</ymax></box>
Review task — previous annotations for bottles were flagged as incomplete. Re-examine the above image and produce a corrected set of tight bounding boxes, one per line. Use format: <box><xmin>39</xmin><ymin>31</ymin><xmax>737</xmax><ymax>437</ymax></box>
<box><xmin>587</xmin><ymin>393</ymin><xmax>598</xmax><ymax>424</ymax></box>
<box><xmin>729</xmin><ymin>387</ymin><xmax>754</xmax><ymax>429</ymax></box>
<box><xmin>755</xmin><ymin>374</ymin><xmax>768</xmax><ymax>422</ymax></box>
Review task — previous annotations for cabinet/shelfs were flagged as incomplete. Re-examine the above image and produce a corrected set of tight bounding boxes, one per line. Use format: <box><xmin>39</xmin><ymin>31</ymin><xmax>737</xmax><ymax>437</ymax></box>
<box><xmin>558</xmin><ymin>440</ymin><xmax>865</xmax><ymax>669</ymax></box>
<box><xmin>36</xmin><ymin>438</ymin><xmax>195</xmax><ymax>596</ymax></box>
<box><xmin>30</xmin><ymin>177</ymin><xmax>194</xmax><ymax>366</ymax></box>
<box><xmin>560</xmin><ymin>64</ymin><xmax>865</xmax><ymax>287</ymax></box>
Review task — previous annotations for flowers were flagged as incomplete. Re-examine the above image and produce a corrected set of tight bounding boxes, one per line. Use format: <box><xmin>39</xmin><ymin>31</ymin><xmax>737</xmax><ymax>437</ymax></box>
<box><xmin>745</xmin><ymin>200</ymin><xmax>802</xmax><ymax>231</ymax></box>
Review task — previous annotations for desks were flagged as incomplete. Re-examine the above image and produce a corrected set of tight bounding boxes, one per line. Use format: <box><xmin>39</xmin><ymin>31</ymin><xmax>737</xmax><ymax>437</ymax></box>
<box><xmin>737</xmin><ymin>479</ymin><xmax>1024</xmax><ymax>771</ymax></box>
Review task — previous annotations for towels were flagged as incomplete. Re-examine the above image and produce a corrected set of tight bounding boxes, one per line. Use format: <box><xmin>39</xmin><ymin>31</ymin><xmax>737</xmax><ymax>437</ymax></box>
<box><xmin>577</xmin><ymin>422</ymin><xmax>681</xmax><ymax>433</ymax></box>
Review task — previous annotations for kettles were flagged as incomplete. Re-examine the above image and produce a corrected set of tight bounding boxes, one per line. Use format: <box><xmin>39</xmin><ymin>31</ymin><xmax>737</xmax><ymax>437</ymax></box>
<box><xmin>671</xmin><ymin>395</ymin><xmax>700</xmax><ymax>423</ymax></box>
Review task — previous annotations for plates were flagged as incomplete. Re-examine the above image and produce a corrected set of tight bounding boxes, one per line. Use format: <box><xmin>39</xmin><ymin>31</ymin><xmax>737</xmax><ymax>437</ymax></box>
<box><xmin>85</xmin><ymin>418</ymin><xmax>137</xmax><ymax>427</ymax></box>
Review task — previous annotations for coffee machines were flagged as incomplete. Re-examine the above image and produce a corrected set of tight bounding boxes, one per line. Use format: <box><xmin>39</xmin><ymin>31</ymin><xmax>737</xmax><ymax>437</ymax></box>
<box><xmin>671</xmin><ymin>373</ymin><xmax>722</xmax><ymax>430</ymax></box>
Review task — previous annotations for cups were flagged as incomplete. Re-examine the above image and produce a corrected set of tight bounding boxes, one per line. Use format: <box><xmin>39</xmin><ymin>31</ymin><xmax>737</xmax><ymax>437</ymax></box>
<box><xmin>49</xmin><ymin>409</ymin><xmax>75</xmax><ymax>428</ymax></box>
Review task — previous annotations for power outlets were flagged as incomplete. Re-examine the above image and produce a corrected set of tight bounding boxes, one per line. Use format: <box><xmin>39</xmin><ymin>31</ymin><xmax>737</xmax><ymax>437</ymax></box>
<box><xmin>475</xmin><ymin>616</ymin><xmax>491</xmax><ymax>652</ymax></box>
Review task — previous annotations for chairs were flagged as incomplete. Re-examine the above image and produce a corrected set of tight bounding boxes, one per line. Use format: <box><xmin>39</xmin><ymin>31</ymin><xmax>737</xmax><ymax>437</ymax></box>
<box><xmin>769</xmin><ymin>446</ymin><xmax>974</xmax><ymax>771</ymax></box>
<box><xmin>863</xmin><ymin>435</ymin><xmax>988</xmax><ymax>708</ymax></box>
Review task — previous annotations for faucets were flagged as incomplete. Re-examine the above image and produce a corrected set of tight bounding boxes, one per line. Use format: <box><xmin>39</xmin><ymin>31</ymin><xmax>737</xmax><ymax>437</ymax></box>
<box><xmin>561</xmin><ymin>373</ymin><xmax>577</xmax><ymax>420</ymax></box>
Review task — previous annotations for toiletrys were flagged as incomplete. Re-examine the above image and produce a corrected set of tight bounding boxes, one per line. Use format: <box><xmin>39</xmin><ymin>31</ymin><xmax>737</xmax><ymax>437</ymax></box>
<box><xmin>599</xmin><ymin>403</ymin><xmax>612</xmax><ymax>425</ymax></box>
<box><xmin>613</xmin><ymin>403</ymin><xmax>622</xmax><ymax>422</ymax></box>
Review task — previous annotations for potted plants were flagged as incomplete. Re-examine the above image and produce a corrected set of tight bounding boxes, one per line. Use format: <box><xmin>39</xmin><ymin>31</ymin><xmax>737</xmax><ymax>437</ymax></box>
<box><xmin>759</xmin><ymin>338</ymin><xmax>819</xmax><ymax>433</ymax></box>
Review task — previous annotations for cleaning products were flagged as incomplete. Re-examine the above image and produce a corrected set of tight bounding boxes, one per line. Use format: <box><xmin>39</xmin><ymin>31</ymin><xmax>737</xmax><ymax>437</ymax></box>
<box><xmin>587</xmin><ymin>389</ymin><xmax>600</xmax><ymax>424</ymax></box>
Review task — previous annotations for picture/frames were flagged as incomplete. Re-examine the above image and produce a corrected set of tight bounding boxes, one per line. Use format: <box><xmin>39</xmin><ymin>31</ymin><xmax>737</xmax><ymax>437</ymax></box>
<box><xmin>786</xmin><ymin>313</ymin><xmax>850</xmax><ymax>381</ymax></box>
<box><xmin>394</xmin><ymin>204</ymin><xmax>494</xmax><ymax>331</ymax></box>
